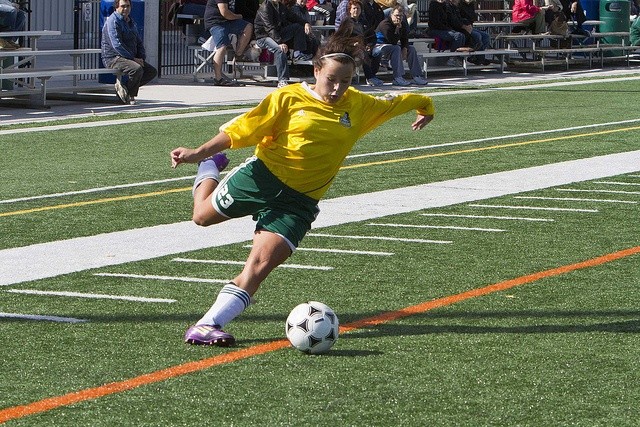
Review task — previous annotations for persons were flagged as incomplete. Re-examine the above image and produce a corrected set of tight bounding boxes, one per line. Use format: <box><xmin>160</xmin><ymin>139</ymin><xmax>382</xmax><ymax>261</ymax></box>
<box><xmin>630</xmin><ymin>14</ymin><xmax>640</xmax><ymax>47</ymax></box>
<box><xmin>396</xmin><ymin>0</ymin><xmax>424</xmax><ymax>39</ymax></box>
<box><xmin>307</xmin><ymin>0</ymin><xmax>335</xmax><ymax>26</ymax></box>
<box><xmin>532</xmin><ymin>0</ymin><xmax>569</xmax><ymax>37</ymax></box>
<box><xmin>561</xmin><ymin>0</ymin><xmax>590</xmax><ymax>35</ymax></box>
<box><xmin>234</xmin><ymin>0</ymin><xmax>259</xmax><ymax>40</ymax></box>
<box><xmin>375</xmin><ymin>6</ymin><xmax>429</xmax><ymax>86</ymax></box>
<box><xmin>0</xmin><ymin>0</ymin><xmax>26</xmax><ymax>50</ymax></box>
<box><xmin>343</xmin><ymin>0</ymin><xmax>383</xmax><ymax>86</ymax></box>
<box><xmin>173</xmin><ymin>0</ymin><xmax>212</xmax><ymax>44</ymax></box>
<box><xmin>428</xmin><ymin>0</ymin><xmax>466</xmax><ymax>67</ymax></box>
<box><xmin>204</xmin><ymin>0</ymin><xmax>254</xmax><ymax>85</ymax></box>
<box><xmin>101</xmin><ymin>0</ymin><xmax>157</xmax><ymax>104</ymax></box>
<box><xmin>450</xmin><ymin>0</ymin><xmax>469</xmax><ymax>66</ymax></box>
<box><xmin>511</xmin><ymin>0</ymin><xmax>550</xmax><ymax>40</ymax></box>
<box><xmin>461</xmin><ymin>0</ymin><xmax>489</xmax><ymax>67</ymax></box>
<box><xmin>254</xmin><ymin>0</ymin><xmax>312</xmax><ymax>89</ymax></box>
<box><xmin>374</xmin><ymin>0</ymin><xmax>398</xmax><ymax>8</ymax></box>
<box><xmin>287</xmin><ymin>0</ymin><xmax>319</xmax><ymax>61</ymax></box>
<box><xmin>170</xmin><ymin>20</ymin><xmax>434</xmax><ymax>344</ymax></box>
<box><xmin>333</xmin><ymin>0</ymin><xmax>350</xmax><ymax>33</ymax></box>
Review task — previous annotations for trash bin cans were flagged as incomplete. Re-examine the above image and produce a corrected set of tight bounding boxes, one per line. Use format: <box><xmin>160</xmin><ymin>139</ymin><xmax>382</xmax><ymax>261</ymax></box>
<box><xmin>600</xmin><ymin>0</ymin><xmax>631</xmax><ymax>57</ymax></box>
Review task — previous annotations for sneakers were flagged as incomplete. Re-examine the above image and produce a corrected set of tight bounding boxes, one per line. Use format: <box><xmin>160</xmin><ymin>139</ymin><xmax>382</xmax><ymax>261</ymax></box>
<box><xmin>393</xmin><ymin>76</ymin><xmax>410</xmax><ymax>86</ymax></box>
<box><xmin>213</xmin><ymin>78</ymin><xmax>231</xmax><ymax>86</ymax></box>
<box><xmin>411</xmin><ymin>76</ymin><xmax>428</xmax><ymax>85</ymax></box>
<box><xmin>292</xmin><ymin>52</ymin><xmax>313</xmax><ymax>65</ymax></box>
<box><xmin>277</xmin><ymin>80</ymin><xmax>289</xmax><ymax>88</ymax></box>
<box><xmin>235</xmin><ymin>53</ymin><xmax>253</xmax><ymax>62</ymax></box>
<box><xmin>128</xmin><ymin>95</ymin><xmax>135</xmax><ymax>104</ymax></box>
<box><xmin>468</xmin><ymin>54</ymin><xmax>490</xmax><ymax>65</ymax></box>
<box><xmin>114</xmin><ymin>78</ymin><xmax>128</xmax><ymax>103</ymax></box>
<box><xmin>365</xmin><ymin>76</ymin><xmax>383</xmax><ymax>87</ymax></box>
<box><xmin>184</xmin><ymin>324</ymin><xmax>235</xmax><ymax>346</ymax></box>
<box><xmin>198</xmin><ymin>151</ymin><xmax>230</xmax><ymax>172</ymax></box>
<box><xmin>446</xmin><ymin>56</ymin><xmax>463</xmax><ymax>66</ymax></box>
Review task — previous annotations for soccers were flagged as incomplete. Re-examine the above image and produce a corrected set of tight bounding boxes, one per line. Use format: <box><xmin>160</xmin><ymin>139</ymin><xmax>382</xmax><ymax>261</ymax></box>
<box><xmin>285</xmin><ymin>301</ymin><xmax>339</xmax><ymax>355</ymax></box>
